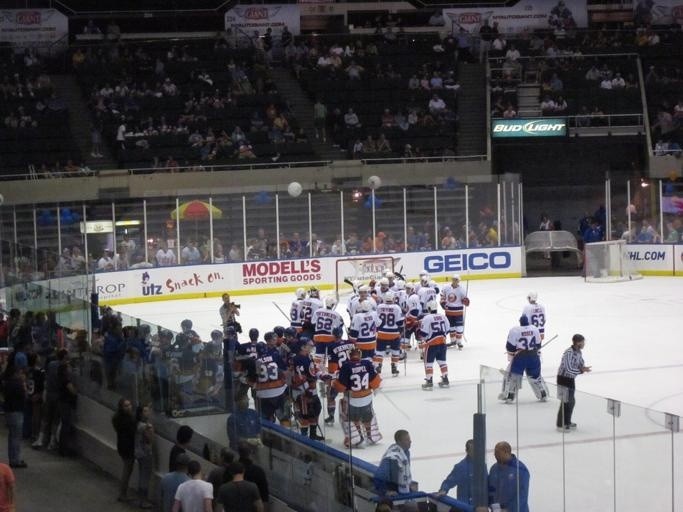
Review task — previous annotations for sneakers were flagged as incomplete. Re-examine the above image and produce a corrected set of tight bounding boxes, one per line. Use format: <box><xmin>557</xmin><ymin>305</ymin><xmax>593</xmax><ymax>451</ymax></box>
<box><xmin>503</xmin><ymin>393</ymin><xmax>514</xmax><ymax>400</ymax></box>
<box><xmin>32</xmin><ymin>440</ymin><xmax>45</xmax><ymax>447</ymax></box>
<box><xmin>117</xmin><ymin>496</ymin><xmax>135</xmax><ymax>502</ymax></box>
<box><xmin>447</xmin><ymin>342</ymin><xmax>463</xmax><ymax>348</ymax></box>
<box><xmin>557</xmin><ymin>423</ymin><xmax>577</xmax><ymax>429</ymax></box>
<box><xmin>47</xmin><ymin>444</ymin><xmax>63</xmax><ymax>451</ymax></box>
<box><xmin>540</xmin><ymin>390</ymin><xmax>547</xmax><ymax>399</ymax></box>
<box><xmin>137</xmin><ymin>498</ymin><xmax>153</xmax><ymax>508</ymax></box>
<box><xmin>9</xmin><ymin>460</ymin><xmax>27</xmax><ymax>468</ymax></box>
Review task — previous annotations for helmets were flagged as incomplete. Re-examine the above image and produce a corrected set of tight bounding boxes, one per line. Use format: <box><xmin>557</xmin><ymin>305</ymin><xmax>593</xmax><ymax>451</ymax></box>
<box><xmin>381</xmin><ymin>271</ymin><xmax>405</xmax><ymax>289</ymax></box>
<box><xmin>426</xmin><ymin>300</ymin><xmax>437</xmax><ymax>312</ymax></box>
<box><xmin>406</xmin><ymin>283</ymin><xmax>415</xmax><ymax>294</ymax></box>
<box><xmin>296</xmin><ymin>287</ymin><xmax>320</xmax><ymax>299</ymax></box>
<box><xmin>451</xmin><ymin>274</ymin><xmax>460</xmax><ymax>282</ymax></box>
<box><xmin>250</xmin><ymin>326</ymin><xmax>297</xmax><ymax>343</ymax></box>
<box><xmin>419</xmin><ymin>270</ymin><xmax>428</xmax><ymax>279</ymax></box>
<box><xmin>420</xmin><ymin>276</ymin><xmax>429</xmax><ymax>286</ymax></box>
<box><xmin>520</xmin><ymin>317</ymin><xmax>529</xmax><ymax>326</ymax></box>
<box><xmin>211</xmin><ymin>325</ymin><xmax>235</xmax><ymax>339</ymax></box>
<box><xmin>161</xmin><ymin>331</ymin><xmax>220</xmax><ymax>359</ymax></box>
<box><xmin>361</xmin><ymin>300</ymin><xmax>371</xmax><ymax>312</ymax></box>
<box><xmin>347</xmin><ymin>348</ymin><xmax>363</xmax><ymax>360</ymax></box>
<box><xmin>527</xmin><ymin>291</ymin><xmax>538</xmax><ymax>302</ymax></box>
<box><xmin>384</xmin><ymin>292</ymin><xmax>395</xmax><ymax>302</ymax></box>
<box><xmin>352</xmin><ymin>279</ymin><xmax>368</xmax><ymax>294</ymax></box>
<box><xmin>333</xmin><ymin>326</ymin><xmax>343</xmax><ymax>337</ymax></box>
<box><xmin>325</xmin><ymin>296</ymin><xmax>337</xmax><ymax>308</ymax></box>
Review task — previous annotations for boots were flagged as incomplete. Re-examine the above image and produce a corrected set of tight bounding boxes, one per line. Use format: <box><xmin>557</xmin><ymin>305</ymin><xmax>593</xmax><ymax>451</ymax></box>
<box><xmin>324</xmin><ymin>416</ymin><xmax>335</xmax><ymax>422</ymax></box>
<box><xmin>309</xmin><ymin>434</ymin><xmax>326</xmax><ymax>441</ymax></box>
<box><xmin>375</xmin><ymin>364</ymin><xmax>382</xmax><ymax>373</ymax></box>
<box><xmin>439</xmin><ymin>378</ymin><xmax>449</xmax><ymax>385</ymax></box>
<box><xmin>392</xmin><ymin>366</ymin><xmax>399</xmax><ymax>373</ymax></box>
<box><xmin>422</xmin><ymin>380</ymin><xmax>433</xmax><ymax>387</ymax></box>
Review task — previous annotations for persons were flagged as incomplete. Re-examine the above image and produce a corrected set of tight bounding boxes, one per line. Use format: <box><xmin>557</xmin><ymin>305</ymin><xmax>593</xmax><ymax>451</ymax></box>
<box><xmin>487</xmin><ymin>441</ymin><xmax>529</xmax><ymax>507</ymax></box>
<box><xmin>1</xmin><ymin>266</ymin><xmax>472</xmax><ymax>511</ymax></box>
<box><xmin>501</xmin><ymin>294</ymin><xmax>550</xmax><ymax>403</ymax></box>
<box><xmin>436</xmin><ymin>438</ymin><xmax>491</xmax><ymax>507</ymax></box>
<box><xmin>1</xmin><ymin>0</ymin><xmax>473</xmax><ymax>288</ymax></box>
<box><xmin>554</xmin><ymin>334</ymin><xmax>592</xmax><ymax>432</ymax></box>
<box><xmin>473</xmin><ymin>1</ymin><xmax>682</xmax><ymax>253</ymax></box>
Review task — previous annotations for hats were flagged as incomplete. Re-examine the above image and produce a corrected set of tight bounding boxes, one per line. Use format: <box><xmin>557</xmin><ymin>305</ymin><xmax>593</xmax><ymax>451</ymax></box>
<box><xmin>572</xmin><ymin>334</ymin><xmax>586</xmax><ymax>343</ymax></box>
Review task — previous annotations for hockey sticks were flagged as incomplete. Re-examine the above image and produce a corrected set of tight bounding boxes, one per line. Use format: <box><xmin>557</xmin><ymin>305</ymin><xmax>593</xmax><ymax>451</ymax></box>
<box><xmin>318</xmin><ymin>424</ymin><xmax>331</xmax><ymax>443</ymax></box>
<box><xmin>395</xmin><ymin>272</ymin><xmax>404</xmax><ymax>280</ymax></box>
<box><xmin>416</xmin><ymin>315</ymin><xmax>425</xmax><ymax>355</ymax></box>
<box><xmin>344</xmin><ymin>279</ymin><xmax>356</xmax><ymax>291</ymax></box>
<box><xmin>401</xmin><ymin>308</ymin><xmax>407</xmax><ymax>377</ymax></box>
<box><xmin>463</xmin><ymin>280</ymin><xmax>468</xmax><ymax>343</ymax></box>
